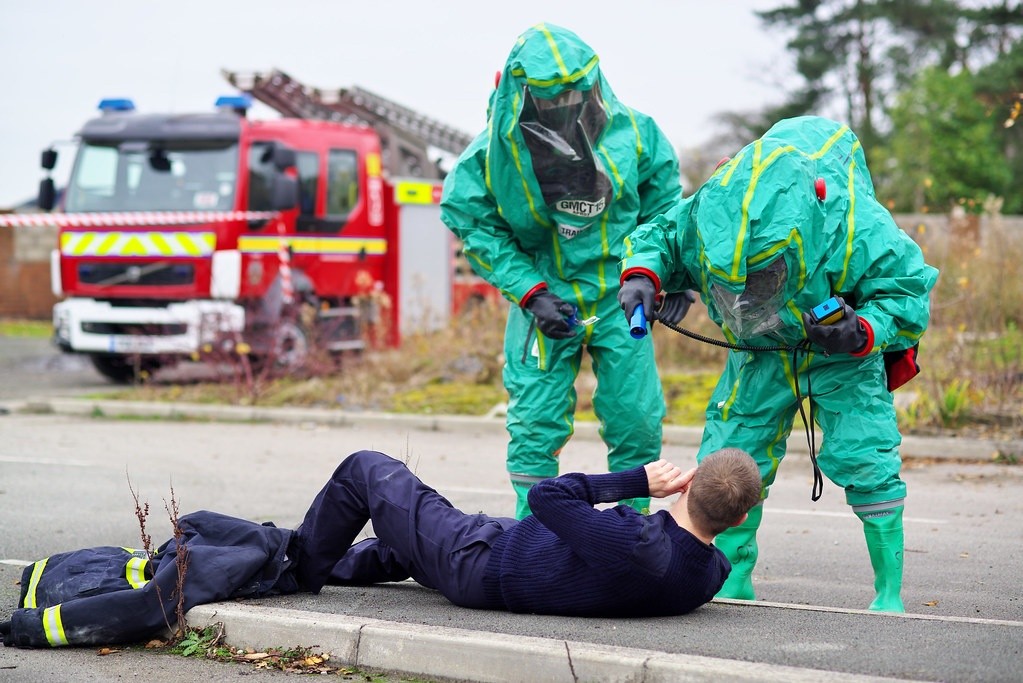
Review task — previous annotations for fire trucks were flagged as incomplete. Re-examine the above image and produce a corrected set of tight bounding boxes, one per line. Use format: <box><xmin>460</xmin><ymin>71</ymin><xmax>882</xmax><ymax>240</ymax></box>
<box><xmin>35</xmin><ymin>69</ymin><xmax>476</xmax><ymax>384</ymax></box>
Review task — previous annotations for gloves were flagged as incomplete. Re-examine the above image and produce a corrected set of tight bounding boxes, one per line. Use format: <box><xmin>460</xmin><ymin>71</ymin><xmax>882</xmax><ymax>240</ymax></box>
<box><xmin>802</xmin><ymin>297</ymin><xmax>868</xmax><ymax>353</ymax></box>
<box><xmin>659</xmin><ymin>290</ymin><xmax>696</xmax><ymax>324</ymax></box>
<box><xmin>524</xmin><ymin>288</ymin><xmax>577</xmax><ymax>339</ymax></box>
<box><xmin>618</xmin><ymin>274</ymin><xmax>661</xmax><ymax>326</ymax></box>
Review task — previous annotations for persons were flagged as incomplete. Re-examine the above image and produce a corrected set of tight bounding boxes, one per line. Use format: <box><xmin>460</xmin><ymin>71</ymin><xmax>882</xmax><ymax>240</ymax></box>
<box><xmin>439</xmin><ymin>25</ymin><xmax>696</xmax><ymax>524</ymax></box>
<box><xmin>261</xmin><ymin>447</ymin><xmax>762</xmax><ymax>620</ymax></box>
<box><xmin>615</xmin><ymin>117</ymin><xmax>939</xmax><ymax>615</ymax></box>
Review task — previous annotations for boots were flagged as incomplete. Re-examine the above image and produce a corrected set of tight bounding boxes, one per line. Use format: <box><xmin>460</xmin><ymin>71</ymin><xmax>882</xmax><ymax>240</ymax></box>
<box><xmin>852</xmin><ymin>498</ymin><xmax>905</xmax><ymax>614</ymax></box>
<box><xmin>714</xmin><ymin>499</ymin><xmax>764</xmax><ymax>601</ymax></box>
<box><xmin>618</xmin><ymin>496</ymin><xmax>651</xmax><ymax>517</ymax></box>
<box><xmin>510</xmin><ymin>474</ymin><xmax>555</xmax><ymax>522</ymax></box>
